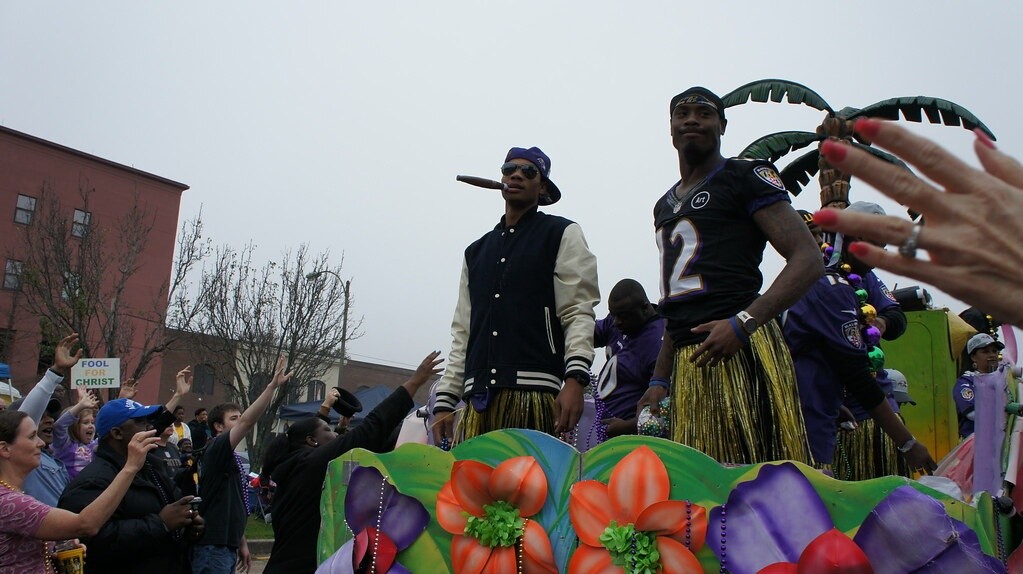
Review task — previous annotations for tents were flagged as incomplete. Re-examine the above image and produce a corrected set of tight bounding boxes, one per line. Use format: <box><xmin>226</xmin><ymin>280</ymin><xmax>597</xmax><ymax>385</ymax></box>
<box><xmin>275</xmin><ymin>382</ymin><xmax>426</xmax><ymax>437</ymax></box>
<box><xmin>0</xmin><ymin>363</ymin><xmax>13</xmax><ymax>404</ymax></box>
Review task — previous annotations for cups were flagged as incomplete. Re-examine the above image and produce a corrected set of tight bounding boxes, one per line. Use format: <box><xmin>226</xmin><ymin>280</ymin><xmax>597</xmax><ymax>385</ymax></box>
<box><xmin>56</xmin><ymin>547</ymin><xmax>84</xmax><ymax>574</ymax></box>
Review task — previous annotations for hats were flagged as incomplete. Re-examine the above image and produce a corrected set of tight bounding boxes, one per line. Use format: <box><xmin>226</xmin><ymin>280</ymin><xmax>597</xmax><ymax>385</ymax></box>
<box><xmin>331</xmin><ymin>387</ymin><xmax>363</xmax><ymax>417</ymax></box>
<box><xmin>885</xmin><ymin>368</ymin><xmax>916</xmax><ymax>405</ymax></box>
<box><xmin>967</xmin><ymin>333</ymin><xmax>1005</xmax><ymax>355</ymax></box>
<box><xmin>670</xmin><ymin>86</ymin><xmax>724</xmax><ymax>122</ymax></box>
<box><xmin>95</xmin><ymin>398</ymin><xmax>163</xmax><ymax>439</ymax></box>
<box><xmin>8</xmin><ymin>397</ymin><xmax>61</xmax><ymax>413</ymax></box>
<box><xmin>504</xmin><ymin>147</ymin><xmax>561</xmax><ymax>206</ymax></box>
<box><xmin>797</xmin><ymin>210</ymin><xmax>815</xmax><ymax>227</ymax></box>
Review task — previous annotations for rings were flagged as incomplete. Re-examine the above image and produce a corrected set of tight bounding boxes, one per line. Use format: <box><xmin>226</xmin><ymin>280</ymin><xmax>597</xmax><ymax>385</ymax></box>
<box><xmin>189</xmin><ymin>510</ymin><xmax>192</xmax><ymax>514</ymax></box>
<box><xmin>898</xmin><ymin>225</ymin><xmax>921</xmax><ymax>260</ymax></box>
<box><xmin>918</xmin><ymin>468</ymin><xmax>922</xmax><ymax>471</ymax></box>
<box><xmin>137</xmin><ymin>438</ymin><xmax>141</xmax><ymax>442</ymax></box>
<box><xmin>925</xmin><ymin>466</ymin><xmax>929</xmax><ymax>470</ymax></box>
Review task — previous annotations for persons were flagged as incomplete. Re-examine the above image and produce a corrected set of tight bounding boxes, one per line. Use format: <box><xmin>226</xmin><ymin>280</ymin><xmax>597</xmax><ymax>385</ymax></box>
<box><xmin>596</xmin><ymin>279</ymin><xmax>666</xmax><ymax>441</ymax></box>
<box><xmin>0</xmin><ymin>331</ymin><xmax>445</xmax><ymax>574</ymax></box>
<box><xmin>637</xmin><ymin>87</ymin><xmax>826</xmax><ymax>468</ymax></box>
<box><xmin>434</xmin><ymin>146</ymin><xmax>600</xmax><ymax>445</ymax></box>
<box><xmin>780</xmin><ymin>200</ymin><xmax>940</xmax><ymax>485</ymax></box>
<box><xmin>811</xmin><ymin>115</ymin><xmax>1023</xmax><ymax>325</ymax></box>
<box><xmin>953</xmin><ymin>332</ymin><xmax>1006</xmax><ymax>442</ymax></box>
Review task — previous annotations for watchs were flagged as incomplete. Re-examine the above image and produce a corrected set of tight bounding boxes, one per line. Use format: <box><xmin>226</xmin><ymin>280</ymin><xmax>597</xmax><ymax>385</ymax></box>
<box><xmin>736</xmin><ymin>311</ymin><xmax>758</xmax><ymax>334</ymax></box>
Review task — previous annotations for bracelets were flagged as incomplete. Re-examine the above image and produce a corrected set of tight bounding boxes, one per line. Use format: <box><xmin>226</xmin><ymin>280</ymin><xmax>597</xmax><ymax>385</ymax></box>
<box><xmin>730</xmin><ymin>317</ymin><xmax>747</xmax><ymax>342</ymax></box>
<box><xmin>648</xmin><ymin>377</ymin><xmax>669</xmax><ymax>389</ymax></box>
<box><xmin>48</xmin><ymin>369</ymin><xmax>64</xmax><ymax>382</ymax></box>
<box><xmin>898</xmin><ymin>436</ymin><xmax>916</xmax><ymax>453</ymax></box>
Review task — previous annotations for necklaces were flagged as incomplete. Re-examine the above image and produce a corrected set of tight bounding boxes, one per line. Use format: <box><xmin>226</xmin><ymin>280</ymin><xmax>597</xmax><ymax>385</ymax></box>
<box><xmin>0</xmin><ymin>480</ymin><xmax>59</xmax><ymax>574</ymax></box>
<box><xmin>587</xmin><ymin>369</ymin><xmax>616</xmax><ymax>450</ymax></box>
<box><xmin>559</xmin><ymin>423</ymin><xmax>579</xmax><ymax>447</ymax></box>
<box><xmin>233</xmin><ymin>451</ymin><xmax>251</xmax><ymax>515</ymax></box>
<box><xmin>831</xmin><ymin>440</ymin><xmax>851</xmax><ymax>480</ymax></box>
<box><xmin>146</xmin><ymin>459</ymin><xmax>179</xmax><ymax>538</ymax></box>
<box><xmin>674</xmin><ymin>177</ymin><xmax>706</xmax><ymax>214</ymax></box>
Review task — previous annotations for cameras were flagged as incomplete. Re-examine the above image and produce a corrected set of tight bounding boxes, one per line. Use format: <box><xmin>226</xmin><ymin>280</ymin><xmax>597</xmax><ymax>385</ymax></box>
<box><xmin>187</xmin><ymin>496</ymin><xmax>203</xmax><ymax>512</ymax></box>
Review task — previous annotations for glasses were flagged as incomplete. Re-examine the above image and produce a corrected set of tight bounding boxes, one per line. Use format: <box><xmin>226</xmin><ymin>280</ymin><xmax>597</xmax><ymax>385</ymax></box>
<box><xmin>55</xmin><ymin>389</ymin><xmax>66</xmax><ymax>393</ymax></box>
<box><xmin>501</xmin><ymin>162</ymin><xmax>542</xmax><ymax>180</ymax></box>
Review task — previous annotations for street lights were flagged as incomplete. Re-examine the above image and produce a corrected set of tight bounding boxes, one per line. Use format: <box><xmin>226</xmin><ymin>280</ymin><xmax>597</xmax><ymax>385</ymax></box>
<box><xmin>305</xmin><ymin>270</ymin><xmax>351</xmax><ymax>388</ymax></box>
